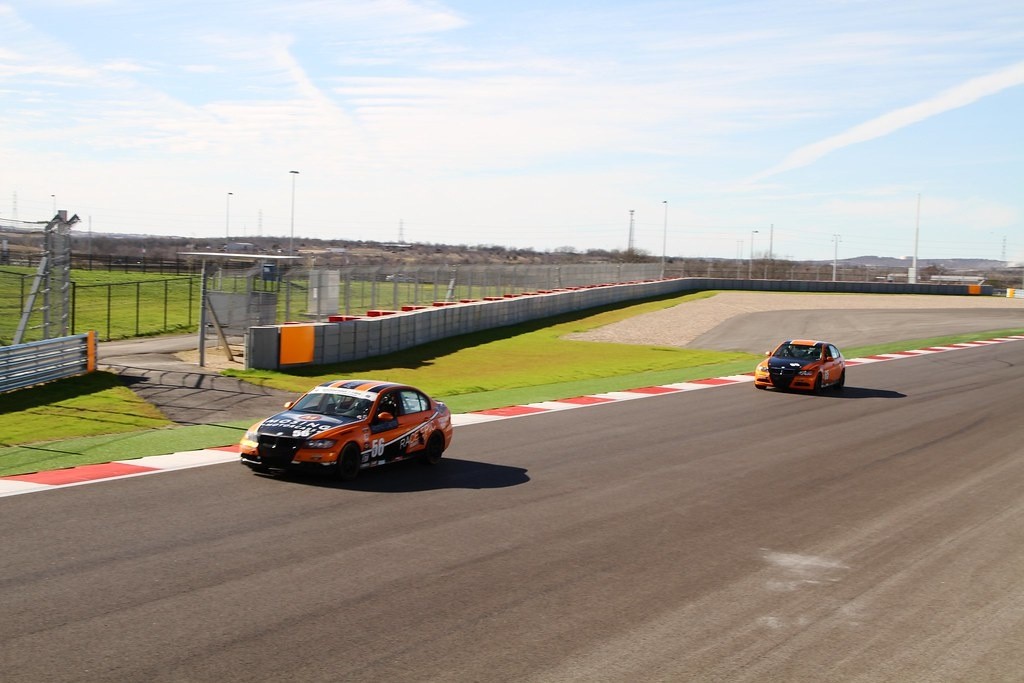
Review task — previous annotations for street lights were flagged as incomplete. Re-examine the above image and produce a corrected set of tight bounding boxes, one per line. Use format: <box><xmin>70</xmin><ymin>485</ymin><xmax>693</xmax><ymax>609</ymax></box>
<box><xmin>749</xmin><ymin>230</ymin><xmax>759</xmax><ymax>279</ymax></box>
<box><xmin>290</xmin><ymin>170</ymin><xmax>299</xmax><ymax>257</ymax></box>
<box><xmin>51</xmin><ymin>194</ymin><xmax>58</xmax><ymax>219</ymax></box>
<box><xmin>662</xmin><ymin>200</ymin><xmax>667</xmax><ymax>277</ymax></box>
<box><xmin>226</xmin><ymin>192</ymin><xmax>234</xmax><ymax>249</ymax></box>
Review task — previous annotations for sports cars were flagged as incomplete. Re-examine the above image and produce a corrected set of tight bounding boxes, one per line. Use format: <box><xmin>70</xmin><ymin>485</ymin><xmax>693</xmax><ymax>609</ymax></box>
<box><xmin>754</xmin><ymin>340</ymin><xmax>846</xmax><ymax>394</ymax></box>
<box><xmin>240</xmin><ymin>378</ymin><xmax>454</xmax><ymax>488</ymax></box>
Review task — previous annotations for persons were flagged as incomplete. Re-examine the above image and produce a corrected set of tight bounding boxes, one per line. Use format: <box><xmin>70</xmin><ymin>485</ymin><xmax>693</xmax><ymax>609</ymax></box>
<box><xmin>819</xmin><ymin>348</ymin><xmax>829</xmax><ymax>357</ymax></box>
<box><xmin>380</xmin><ymin>393</ymin><xmax>397</xmax><ymax>414</ymax></box>
